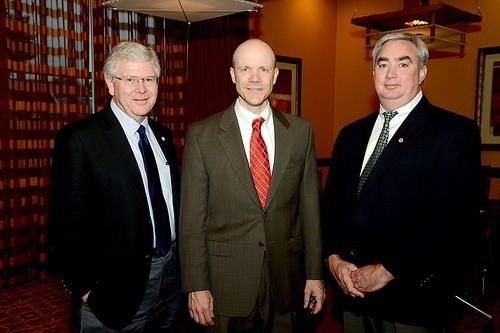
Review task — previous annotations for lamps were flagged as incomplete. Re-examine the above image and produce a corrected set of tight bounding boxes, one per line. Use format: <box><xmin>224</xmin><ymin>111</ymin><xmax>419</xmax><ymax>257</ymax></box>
<box><xmin>102</xmin><ymin>0</ymin><xmax>263</xmax><ymax>23</ymax></box>
<box><xmin>351</xmin><ymin>0</ymin><xmax>482</xmax><ymax>59</ymax></box>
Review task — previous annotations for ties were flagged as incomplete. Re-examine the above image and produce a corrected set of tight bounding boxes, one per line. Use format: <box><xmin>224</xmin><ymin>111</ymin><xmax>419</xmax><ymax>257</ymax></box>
<box><xmin>249</xmin><ymin>116</ymin><xmax>271</xmax><ymax>251</ymax></box>
<box><xmin>137</xmin><ymin>125</ymin><xmax>173</xmax><ymax>257</ymax></box>
<box><xmin>357</xmin><ymin>111</ymin><xmax>398</xmax><ymax>196</ymax></box>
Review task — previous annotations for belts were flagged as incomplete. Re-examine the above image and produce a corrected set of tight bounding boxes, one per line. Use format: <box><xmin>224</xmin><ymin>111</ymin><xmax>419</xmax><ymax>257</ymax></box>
<box><xmin>153</xmin><ymin>241</ymin><xmax>176</xmax><ymax>258</ymax></box>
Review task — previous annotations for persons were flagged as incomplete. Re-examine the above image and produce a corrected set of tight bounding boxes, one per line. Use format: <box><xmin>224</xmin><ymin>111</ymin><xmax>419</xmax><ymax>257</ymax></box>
<box><xmin>321</xmin><ymin>33</ymin><xmax>481</xmax><ymax>333</ymax></box>
<box><xmin>179</xmin><ymin>38</ymin><xmax>326</xmax><ymax>333</ymax></box>
<box><xmin>49</xmin><ymin>41</ymin><xmax>181</xmax><ymax>333</ymax></box>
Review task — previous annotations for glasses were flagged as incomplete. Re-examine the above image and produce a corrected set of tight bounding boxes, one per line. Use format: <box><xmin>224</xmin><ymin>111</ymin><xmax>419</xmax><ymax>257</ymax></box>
<box><xmin>115</xmin><ymin>76</ymin><xmax>159</xmax><ymax>86</ymax></box>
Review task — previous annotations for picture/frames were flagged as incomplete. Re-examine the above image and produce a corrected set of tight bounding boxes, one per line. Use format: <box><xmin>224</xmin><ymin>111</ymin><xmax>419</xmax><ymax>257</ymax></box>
<box><xmin>268</xmin><ymin>55</ymin><xmax>302</xmax><ymax>117</ymax></box>
<box><xmin>474</xmin><ymin>46</ymin><xmax>500</xmax><ymax>151</ymax></box>
